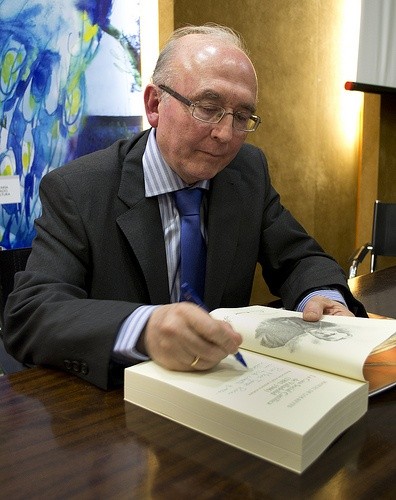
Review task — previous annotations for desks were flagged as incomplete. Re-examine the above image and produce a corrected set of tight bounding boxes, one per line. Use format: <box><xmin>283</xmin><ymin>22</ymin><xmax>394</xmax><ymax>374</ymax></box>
<box><xmin>0</xmin><ymin>265</ymin><xmax>396</xmax><ymax>500</ymax></box>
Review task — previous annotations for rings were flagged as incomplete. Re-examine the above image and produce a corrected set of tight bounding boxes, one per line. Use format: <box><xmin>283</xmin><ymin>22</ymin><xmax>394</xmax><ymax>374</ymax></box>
<box><xmin>189</xmin><ymin>357</ymin><xmax>200</xmax><ymax>368</ymax></box>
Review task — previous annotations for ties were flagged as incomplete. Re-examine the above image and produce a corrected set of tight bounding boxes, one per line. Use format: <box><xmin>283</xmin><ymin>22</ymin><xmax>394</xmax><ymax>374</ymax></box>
<box><xmin>170</xmin><ymin>187</ymin><xmax>207</xmax><ymax>305</ymax></box>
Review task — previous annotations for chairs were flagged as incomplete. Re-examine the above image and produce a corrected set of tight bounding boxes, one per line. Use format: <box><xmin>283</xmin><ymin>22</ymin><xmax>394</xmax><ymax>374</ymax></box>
<box><xmin>348</xmin><ymin>200</ymin><xmax>396</xmax><ymax>279</ymax></box>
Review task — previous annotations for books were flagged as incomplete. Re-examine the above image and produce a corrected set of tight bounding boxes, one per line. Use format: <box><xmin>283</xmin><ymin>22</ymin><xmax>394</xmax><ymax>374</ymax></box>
<box><xmin>124</xmin><ymin>305</ymin><xmax>396</xmax><ymax>474</ymax></box>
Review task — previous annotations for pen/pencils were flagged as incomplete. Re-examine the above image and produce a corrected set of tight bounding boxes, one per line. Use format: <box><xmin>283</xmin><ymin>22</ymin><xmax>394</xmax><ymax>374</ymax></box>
<box><xmin>182</xmin><ymin>283</ymin><xmax>248</xmax><ymax>368</ymax></box>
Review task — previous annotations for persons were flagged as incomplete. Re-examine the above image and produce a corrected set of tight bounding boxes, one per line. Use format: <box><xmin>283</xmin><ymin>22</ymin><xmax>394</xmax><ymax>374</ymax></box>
<box><xmin>3</xmin><ymin>25</ymin><xmax>369</xmax><ymax>390</ymax></box>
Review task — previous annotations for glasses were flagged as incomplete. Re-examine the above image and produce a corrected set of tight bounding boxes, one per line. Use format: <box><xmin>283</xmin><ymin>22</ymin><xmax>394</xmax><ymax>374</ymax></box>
<box><xmin>158</xmin><ymin>83</ymin><xmax>262</xmax><ymax>134</ymax></box>
